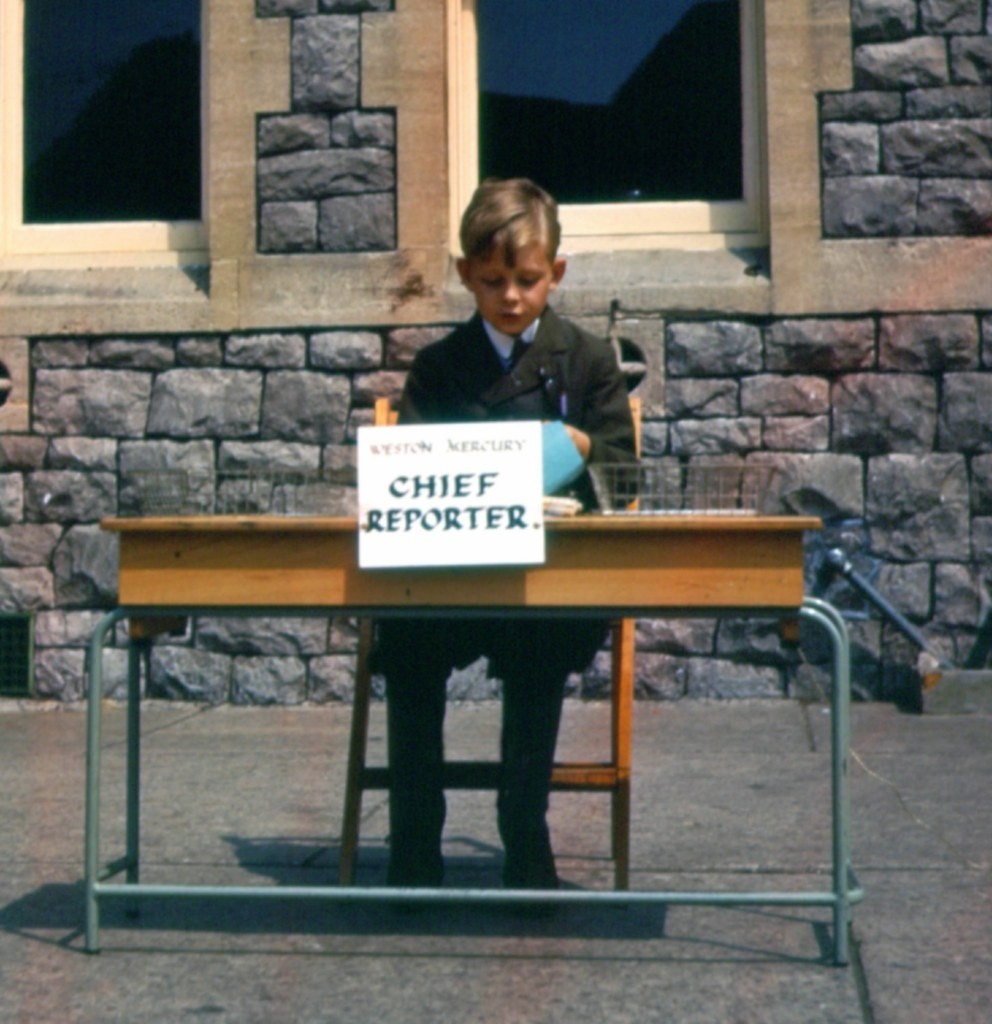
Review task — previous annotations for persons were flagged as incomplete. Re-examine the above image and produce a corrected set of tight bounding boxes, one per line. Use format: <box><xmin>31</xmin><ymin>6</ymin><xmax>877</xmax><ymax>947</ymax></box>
<box><xmin>370</xmin><ymin>177</ymin><xmax>646</xmax><ymax>910</ymax></box>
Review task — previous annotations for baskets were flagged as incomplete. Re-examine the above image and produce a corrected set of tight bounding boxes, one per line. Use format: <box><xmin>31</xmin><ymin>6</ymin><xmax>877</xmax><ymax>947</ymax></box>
<box><xmin>588</xmin><ymin>462</ymin><xmax>762</xmax><ymax>516</ymax></box>
<box><xmin>121</xmin><ymin>468</ymin><xmax>304</xmax><ymax>518</ymax></box>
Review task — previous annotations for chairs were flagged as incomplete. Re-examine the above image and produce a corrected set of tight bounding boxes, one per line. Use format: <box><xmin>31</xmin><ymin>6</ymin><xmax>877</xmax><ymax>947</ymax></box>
<box><xmin>333</xmin><ymin>399</ymin><xmax>645</xmax><ymax>909</ymax></box>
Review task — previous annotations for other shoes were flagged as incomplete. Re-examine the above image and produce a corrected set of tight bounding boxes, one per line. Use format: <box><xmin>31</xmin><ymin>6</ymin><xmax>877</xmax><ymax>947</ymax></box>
<box><xmin>386</xmin><ymin>839</ymin><xmax>443</xmax><ymax>914</ymax></box>
<box><xmin>503</xmin><ymin>836</ymin><xmax>559</xmax><ymax>919</ymax></box>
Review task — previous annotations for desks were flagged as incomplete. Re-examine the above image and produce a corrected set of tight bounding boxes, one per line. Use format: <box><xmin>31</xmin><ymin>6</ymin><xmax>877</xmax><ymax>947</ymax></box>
<box><xmin>79</xmin><ymin>515</ymin><xmax>863</xmax><ymax>970</ymax></box>
